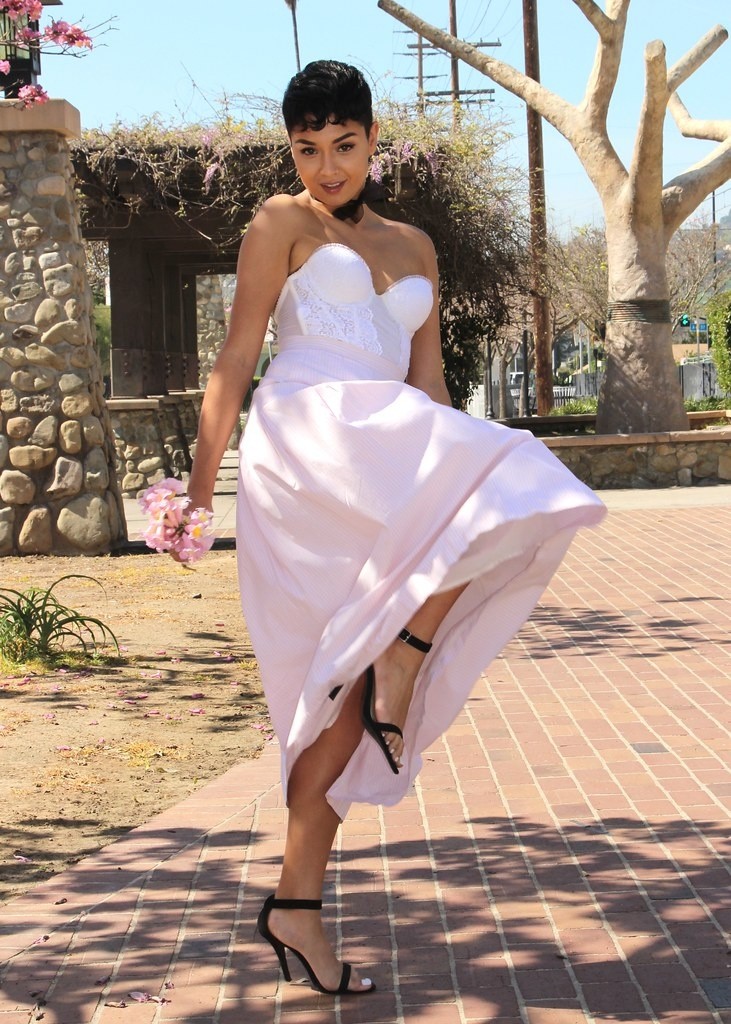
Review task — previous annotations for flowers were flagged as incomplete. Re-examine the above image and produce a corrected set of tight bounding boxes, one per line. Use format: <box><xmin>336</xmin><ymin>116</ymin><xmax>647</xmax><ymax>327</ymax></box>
<box><xmin>134</xmin><ymin>476</ymin><xmax>216</xmax><ymax>571</ymax></box>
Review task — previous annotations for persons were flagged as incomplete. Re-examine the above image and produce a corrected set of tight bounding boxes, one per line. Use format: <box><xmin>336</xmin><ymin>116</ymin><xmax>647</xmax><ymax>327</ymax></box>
<box><xmin>164</xmin><ymin>60</ymin><xmax>607</xmax><ymax>997</ymax></box>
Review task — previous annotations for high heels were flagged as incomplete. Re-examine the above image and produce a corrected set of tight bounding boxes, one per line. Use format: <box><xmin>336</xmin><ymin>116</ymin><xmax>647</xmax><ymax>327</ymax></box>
<box><xmin>329</xmin><ymin>623</ymin><xmax>431</xmax><ymax>776</ymax></box>
<box><xmin>257</xmin><ymin>894</ymin><xmax>377</xmax><ymax>995</ymax></box>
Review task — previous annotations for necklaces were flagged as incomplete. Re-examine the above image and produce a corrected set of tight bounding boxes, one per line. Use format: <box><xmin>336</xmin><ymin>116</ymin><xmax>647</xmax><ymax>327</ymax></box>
<box><xmin>310</xmin><ymin>172</ymin><xmax>391</xmax><ymax>225</ymax></box>
